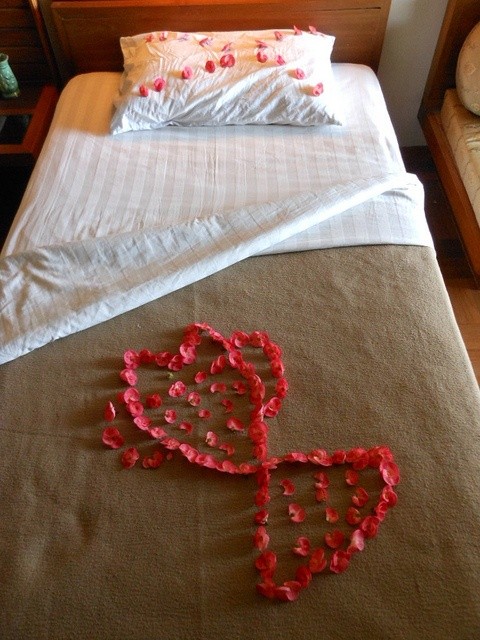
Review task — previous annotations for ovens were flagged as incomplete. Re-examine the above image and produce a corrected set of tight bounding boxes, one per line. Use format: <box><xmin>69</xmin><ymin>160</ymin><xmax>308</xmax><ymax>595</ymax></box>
<box><xmin>0</xmin><ymin>1</ymin><xmax>480</xmax><ymax>638</ymax></box>
<box><xmin>416</xmin><ymin>0</ymin><xmax>479</xmax><ymax>290</ymax></box>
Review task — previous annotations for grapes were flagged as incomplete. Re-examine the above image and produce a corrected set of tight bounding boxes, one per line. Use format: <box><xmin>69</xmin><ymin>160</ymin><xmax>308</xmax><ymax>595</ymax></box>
<box><xmin>0</xmin><ymin>84</ymin><xmax>61</xmax><ymax>171</ymax></box>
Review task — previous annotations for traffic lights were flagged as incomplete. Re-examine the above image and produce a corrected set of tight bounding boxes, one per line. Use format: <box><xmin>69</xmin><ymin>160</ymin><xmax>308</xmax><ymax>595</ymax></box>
<box><xmin>111</xmin><ymin>27</ymin><xmax>342</xmax><ymax>136</ymax></box>
